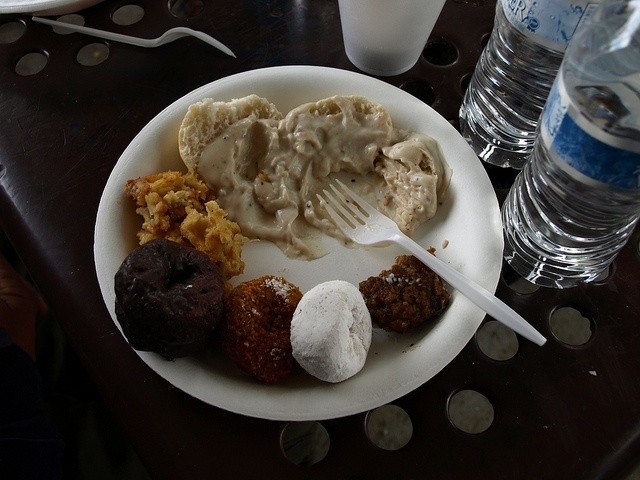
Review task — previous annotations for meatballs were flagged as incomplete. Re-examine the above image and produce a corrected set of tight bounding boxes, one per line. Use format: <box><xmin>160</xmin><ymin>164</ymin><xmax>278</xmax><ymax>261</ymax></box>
<box><xmin>291</xmin><ymin>280</ymin><xmax>372</xmax><ymax>383</ymax></box>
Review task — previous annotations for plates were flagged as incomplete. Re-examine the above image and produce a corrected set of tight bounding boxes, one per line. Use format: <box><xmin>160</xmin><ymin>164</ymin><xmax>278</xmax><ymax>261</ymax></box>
<box><xmin>94</xmin><ymin>65</ymin><xmax>500</xmax><ymax>421</ymax></box>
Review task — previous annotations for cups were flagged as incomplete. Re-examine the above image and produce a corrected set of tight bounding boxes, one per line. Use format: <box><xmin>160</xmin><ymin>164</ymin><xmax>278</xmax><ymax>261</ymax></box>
<box><xmin>338</xmin><ymin>0</ymin><xmax>445</xmax><ymax>75</ymax></box>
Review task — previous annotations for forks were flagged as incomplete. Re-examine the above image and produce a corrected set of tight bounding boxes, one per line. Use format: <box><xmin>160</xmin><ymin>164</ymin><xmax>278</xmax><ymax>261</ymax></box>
<box><xmin>31</xmin><ymin>13</ymin><xmax>238</xmax><ymax>62</ymax></box>
<box><xmin>315</xmin><ymin>178</ymin><xmax>548</xmax><ymax>364</ymax></box>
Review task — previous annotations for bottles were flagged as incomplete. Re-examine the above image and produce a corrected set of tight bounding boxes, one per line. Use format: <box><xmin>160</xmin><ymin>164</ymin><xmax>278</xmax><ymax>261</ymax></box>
<box><xmin>459</xmin><ymin>0</ymin><xmax>588</xmax><ymax>171</ymax></box>
<box><xmin>500</xmin><ymin>0</ymin><xmax>640</xmax><ymax>289</ymax></box>
